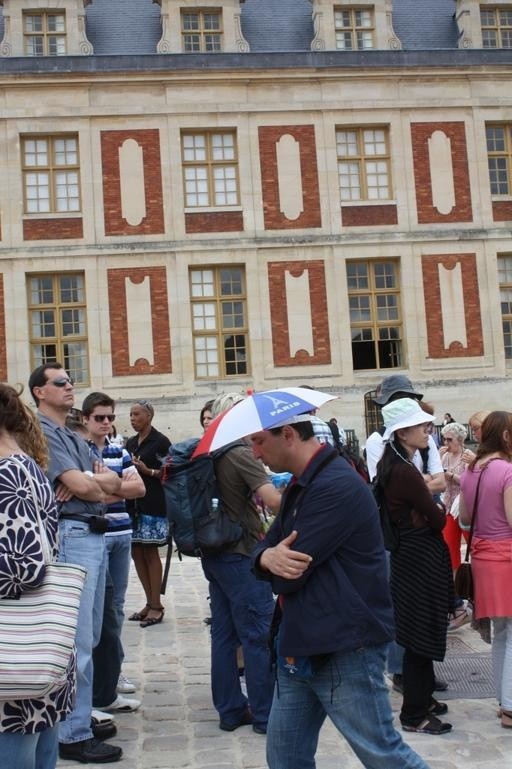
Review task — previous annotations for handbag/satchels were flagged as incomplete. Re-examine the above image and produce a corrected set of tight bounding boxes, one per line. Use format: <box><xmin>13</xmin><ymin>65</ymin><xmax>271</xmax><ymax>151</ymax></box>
<box><xmin>455</xmin><ymin>562</ymin><xmax>473</xmax><ymax>599</ymax></box>
<box><xmin>0</xmin><ymin>456</ymin><xmax>88</xmax><ymax>701</ymax></box>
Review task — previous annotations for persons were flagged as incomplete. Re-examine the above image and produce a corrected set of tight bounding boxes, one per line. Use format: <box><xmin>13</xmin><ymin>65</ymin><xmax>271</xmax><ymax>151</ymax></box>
<box><xmin>1</xmin><ymin>358</ymin><xmax>512</xmax><ymax>769</ymax></box>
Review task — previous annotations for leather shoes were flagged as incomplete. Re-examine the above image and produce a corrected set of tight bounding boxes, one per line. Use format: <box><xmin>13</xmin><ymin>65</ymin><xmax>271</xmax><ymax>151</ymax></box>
<box><xmin>59</xmin><ymin>737</ymin><xmax>122</xmax><ymax>762</ymax></box>
<box><xmin>90</xmin><ymin>718</ymin><xmax>117</xmax><ymax>738</ymax></box>
<box><xmin>219</xmin><ymin>706</ymin><xmax>253</xmax><ymax>731</ymax></box>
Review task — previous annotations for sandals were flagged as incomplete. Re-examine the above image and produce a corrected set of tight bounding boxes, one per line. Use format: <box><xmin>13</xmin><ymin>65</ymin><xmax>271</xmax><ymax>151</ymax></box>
<box><xmin>139</xmin><ymin>604</ymin><xmax>164</xmax><ymax>628</ymax></box>
<box><xmin>501</xmin><ymin>711</ymin><xmax>512</xmax><ymax>728</ymax></box>
<box><xmin>128</xmin><ymin>603</ymin><xmax>151</xmax><ymax>620</ymax></box>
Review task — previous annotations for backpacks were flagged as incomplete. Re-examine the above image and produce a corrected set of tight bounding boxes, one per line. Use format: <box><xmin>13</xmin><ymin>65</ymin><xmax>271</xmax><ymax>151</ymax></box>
<box><xmin>366</xmin><ymin>457</ymin><xmax>425</xmax><ymax>552</ymax></box>
<box><xmin>159</xmin><ymin>437</ymin><xmax>253</xmax><ymax>561</ymax></box>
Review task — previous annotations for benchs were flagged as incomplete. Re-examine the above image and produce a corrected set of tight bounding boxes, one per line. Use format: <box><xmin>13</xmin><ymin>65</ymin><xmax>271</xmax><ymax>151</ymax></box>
<box><xmin>345</xmin><ymin>429</ymin><xmax>359</xmax><ymax>450</ymax></box>
<box><xmin>436</xmin><ymin>423</ymin><xmax>480</xmax><ymax>444</ymax></box>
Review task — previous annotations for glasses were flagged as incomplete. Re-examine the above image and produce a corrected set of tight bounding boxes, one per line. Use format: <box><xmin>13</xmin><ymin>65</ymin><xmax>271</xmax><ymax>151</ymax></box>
<box><xmin>39</xmin><ymin>376</ymin><xmax>74</xmax><ymax>385</ymax></box>
<box><xmin>443</xmin><ymin>436</ymin><xmax>453</xmax><ymax>442</ymax></box>
<box><xmin>88</xmin><ymin>413</ymin><xmax>115</xmax><ymax>421</ymax></box>
<box><xmin>135</xmin><ymin>398</ymin><xmax>151</xmax><ymax>411</ymax></box>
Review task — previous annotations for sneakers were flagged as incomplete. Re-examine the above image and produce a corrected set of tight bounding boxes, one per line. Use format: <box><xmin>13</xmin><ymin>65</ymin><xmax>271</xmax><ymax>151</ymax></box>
<box><xmin>402</xmin><ymin>712</ymin><xmax>453</xmax><ymax>734</ymax></box>
<box><xmin>393</xmin><ymin>673</ymin><xmax>404</xmax><ymax>693</ymax></box>
<box><xmin>433</xmin><ymin>674</ymin><xmax>448</xmax><ymax>692</ymax></box>
<box><xmin>93</xmin><ymin>694</ymin><xmax>141</xmax><ymax>713</ymax></box>
<box><xmin>426</xmin><ymin>700</ymin><xmax>448</xmax><ymax>715</ymax></box>
<box><xmin>116</xmin><ymin>671</ymin><xmax>137</xmax><ymax>695</ymax></box>
<box><xmin>91</xmin><ymin>708</ymin><xmax>115</xmax><ymax>725</ymax></box>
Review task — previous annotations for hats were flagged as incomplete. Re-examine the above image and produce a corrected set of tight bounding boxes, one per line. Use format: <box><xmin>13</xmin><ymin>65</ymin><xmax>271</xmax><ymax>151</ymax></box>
<box><xmin>381</xmin><ymin>396</ymin><xmax>437</xmax><ymax>444</ymax></box>
<box><xmin>372</xmin><ymin>372</ymin><xmax>424</xmax><ymax>405</ymax></box>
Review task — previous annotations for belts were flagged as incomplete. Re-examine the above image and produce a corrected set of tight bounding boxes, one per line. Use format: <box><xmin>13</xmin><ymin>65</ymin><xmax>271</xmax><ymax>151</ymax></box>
<box><xmin>59</xmin><ymin>512</ymin><xmax>91</xmax><ymax>523</ymax></box>
